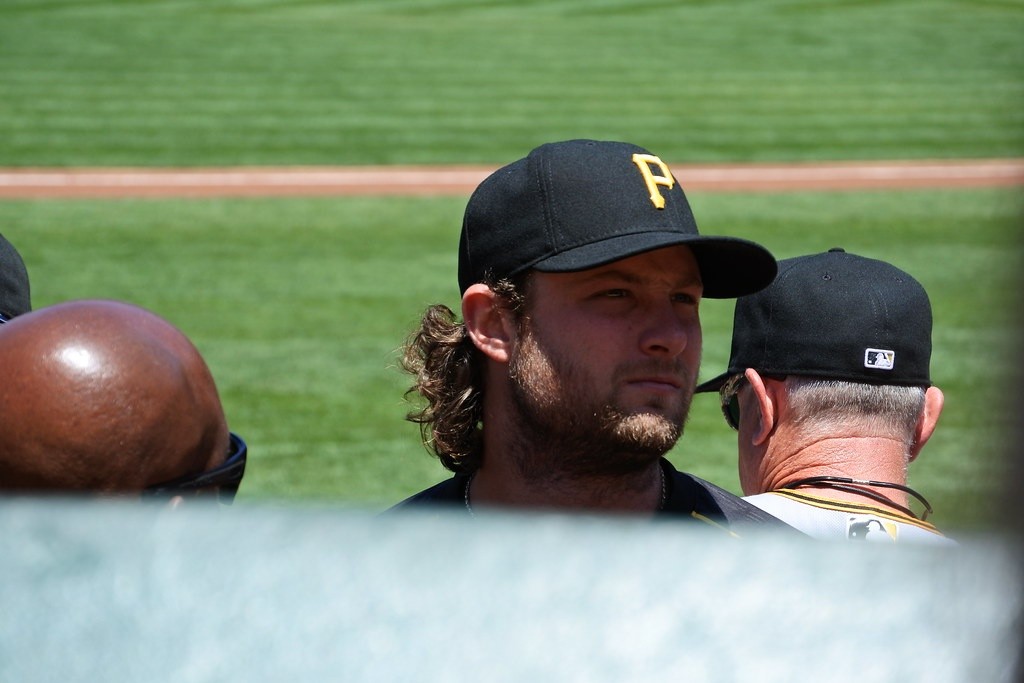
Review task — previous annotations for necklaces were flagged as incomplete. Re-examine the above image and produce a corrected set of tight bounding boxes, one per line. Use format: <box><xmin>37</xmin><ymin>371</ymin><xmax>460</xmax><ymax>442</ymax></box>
<box><xmin>779</xmin><ymin>476</ymin><xmax>934</xmax><ymax>521</ymax></box>
<box><xmin>465</xmin><ymin>466</ymin><xmax>666</xmax><ymax>519</ymax></box>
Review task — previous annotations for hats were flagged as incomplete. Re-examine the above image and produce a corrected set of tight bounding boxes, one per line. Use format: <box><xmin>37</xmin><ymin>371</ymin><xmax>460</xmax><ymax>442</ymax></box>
<box><xmin>1</xmin><ymin>235</ymin><xmax>32</xmax><ymax>320</ymax></box>
<box><xmin>458</xmin><ymin>136</ymin><xmax>778</xmax><ymax>301</ymax></box>
<box><xmin>693</xmin><ymin>247</ymin><xmax>935</xmax><ymax>393</ymax></box>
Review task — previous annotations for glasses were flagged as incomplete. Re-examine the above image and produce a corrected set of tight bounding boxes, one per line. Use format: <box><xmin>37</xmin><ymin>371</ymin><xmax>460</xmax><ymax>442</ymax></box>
<box><xmin>719</xmin><ymin>369</ymin><xmax>786</xmax><ymax>431</ymax></box>
<box><xmin>142</xmin><ymin>433</ymin><xmax>249</xmax><ymax>507</ymax></box>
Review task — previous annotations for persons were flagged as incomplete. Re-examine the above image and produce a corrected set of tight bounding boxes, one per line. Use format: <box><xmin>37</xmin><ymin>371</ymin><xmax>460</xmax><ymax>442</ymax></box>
<box><xmin>695</xmin><ymin>247</ymin><xmax>957</xmax><ymax>546</ymax></box>
<box><xmin>0</xmin><ymin>301</ymin><xmax>248</xmax><ymax>505</ymax></box>
<box><xmin>380</xmin><ymin>141</ymin><xmax>805</xmax><ymax>533</ymax></box>
